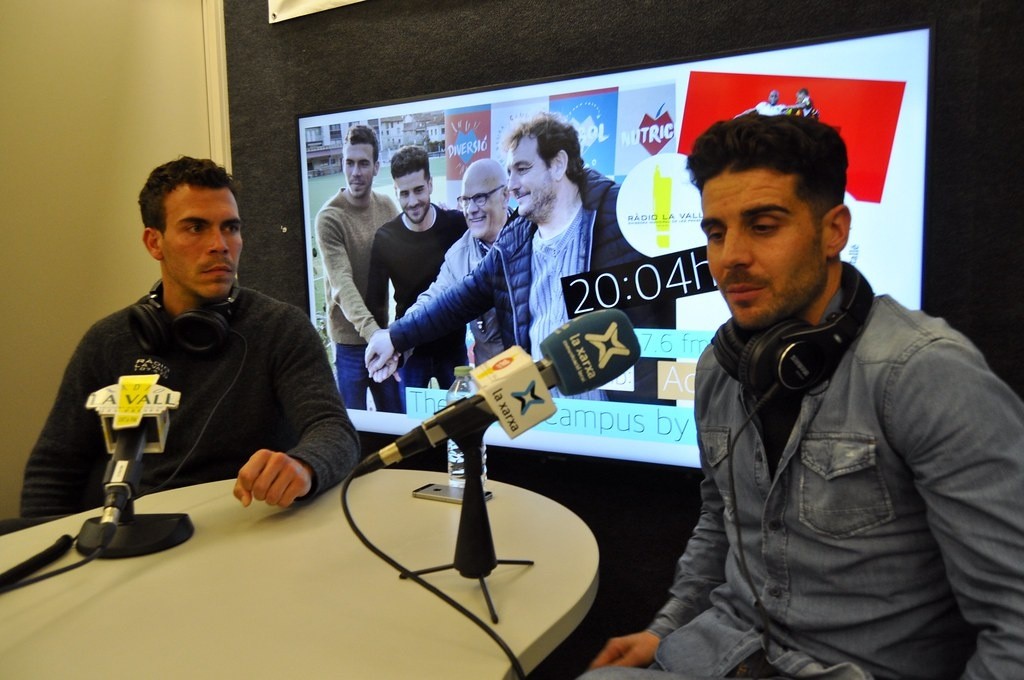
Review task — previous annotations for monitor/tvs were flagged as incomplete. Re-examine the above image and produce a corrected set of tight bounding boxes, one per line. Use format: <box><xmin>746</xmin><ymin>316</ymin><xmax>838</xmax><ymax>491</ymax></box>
<box><xmin>294</xmin><ymin>21</ymin><xmax>938</xmax><ymax>487</ymax></box>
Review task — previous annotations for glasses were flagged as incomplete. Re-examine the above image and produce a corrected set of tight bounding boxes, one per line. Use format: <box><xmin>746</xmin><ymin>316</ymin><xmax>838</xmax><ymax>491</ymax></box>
<box><xmin>456</xmin><ymin>185</ymin><xmax>505</xmax><ymax>209</ymax></box>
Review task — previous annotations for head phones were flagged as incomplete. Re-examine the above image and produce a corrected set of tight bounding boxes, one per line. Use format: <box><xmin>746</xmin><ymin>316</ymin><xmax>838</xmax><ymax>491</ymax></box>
<box><xmin>131</xmin><ymin>274</ymin><xmax>245</xmax><ymax>358</ymax></box>
<box><xmin>708</xmin><ymin>262</ymin><xmax>875</xmax><ymax>405</ymax></box>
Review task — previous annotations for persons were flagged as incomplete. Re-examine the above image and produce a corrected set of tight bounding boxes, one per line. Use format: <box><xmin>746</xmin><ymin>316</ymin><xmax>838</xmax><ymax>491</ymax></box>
<box><xmin>20</xmin><ymin>156</ymin><xmax>362</xmax><ymax>523</ymax></box>
<box><xmin>575</xmin><ymin>115</ymin><xmax>1024</xmax><ymax>680</ymax></box>
<box><xmin>314</xmin><ymin>116</ymin><xmax>677</xmax><ymax>415</ymax></box>
<box><xmin>733</xmin><ymin>87</ymin><xmax>819</xmax><ymax>121</ymax></box>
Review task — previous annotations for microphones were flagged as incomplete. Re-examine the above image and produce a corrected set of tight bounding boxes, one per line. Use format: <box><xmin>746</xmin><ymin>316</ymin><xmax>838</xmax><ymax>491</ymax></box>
<box><xmin>86</xmin><ymin>357</ymin><xmax>181</xmax><ymax>547</ymax></box>
<box><xmin>351</xmin><ymin>310</ymin><xmax>641</xmax><ymax>477</ymax></box>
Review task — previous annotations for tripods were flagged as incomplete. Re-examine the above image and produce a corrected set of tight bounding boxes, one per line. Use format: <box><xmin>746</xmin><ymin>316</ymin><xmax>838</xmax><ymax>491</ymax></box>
<box><xmin>399</xmin><ymin>398</ymin><xmax>535</xmax><ymax>625</ymax></box>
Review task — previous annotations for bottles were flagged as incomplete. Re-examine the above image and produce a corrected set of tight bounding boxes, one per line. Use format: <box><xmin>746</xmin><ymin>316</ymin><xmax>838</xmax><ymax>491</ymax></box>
<box><xmin>446</xmin><ymin>365</ymin><xmax>488</xmax><ymax>487</ymax></box>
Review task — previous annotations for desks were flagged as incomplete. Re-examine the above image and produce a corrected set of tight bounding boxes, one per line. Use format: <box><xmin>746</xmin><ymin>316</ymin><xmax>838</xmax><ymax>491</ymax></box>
<box><xmin>0</xmin><ymin>460</ymin><xmax>601</xmax><ymax>678</ymax></box>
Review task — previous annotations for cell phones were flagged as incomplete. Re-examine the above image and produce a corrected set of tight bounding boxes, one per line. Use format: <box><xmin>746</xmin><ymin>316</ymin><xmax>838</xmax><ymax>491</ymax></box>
<box><xmin>412</xmin><ymin>483</ymin><xmax>493</xmax><ymax>504</ymax></box>
